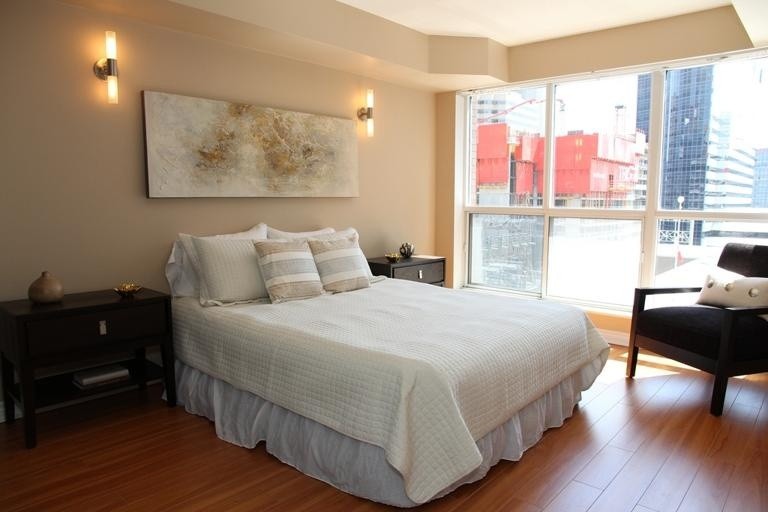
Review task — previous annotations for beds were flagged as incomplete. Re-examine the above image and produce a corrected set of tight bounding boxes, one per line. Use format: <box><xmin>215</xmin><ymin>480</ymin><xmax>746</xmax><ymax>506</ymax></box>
<box><xmin>164</xmin><ymin>222</ymin><xmax>612</xmax><ymax>509</ymax></box>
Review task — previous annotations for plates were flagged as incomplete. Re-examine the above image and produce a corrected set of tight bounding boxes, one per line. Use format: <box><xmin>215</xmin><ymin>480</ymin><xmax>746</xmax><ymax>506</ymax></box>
<box><xmin>385</xmin><ymin>256</ymin><xmax>401</xmax><ymax>262</ymax></box>
<box><xmin>113</xmin><ymin>288</ymin><xmax>142</xmax><ymax>296</ymax></box>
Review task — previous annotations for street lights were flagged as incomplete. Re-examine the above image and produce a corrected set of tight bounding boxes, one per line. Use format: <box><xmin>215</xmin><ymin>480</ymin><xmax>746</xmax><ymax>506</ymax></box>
<box><xmin>672</xmin><ymin>192</ymin><xmax>685</xmax><ymax>270</ymax></box>
<box><xmin>514</xmin><ymin>159</ymin><xmax>538</xmax><ymax>207</ymax></box>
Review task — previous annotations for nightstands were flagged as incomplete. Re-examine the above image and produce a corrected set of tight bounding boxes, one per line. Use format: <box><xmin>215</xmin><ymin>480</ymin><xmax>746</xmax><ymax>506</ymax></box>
<box><xmin>367</xmin><ymin>255</ymin><xmax>446</xmax><ymax>288</ymax></box>
<box><xmin>0</xmin><ymin>284</ymin><xmax>176</xmax><ymax>450</ymax></box>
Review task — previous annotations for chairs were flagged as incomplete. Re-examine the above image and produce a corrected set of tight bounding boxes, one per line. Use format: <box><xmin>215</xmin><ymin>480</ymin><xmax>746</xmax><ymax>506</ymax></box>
<box><xmin>626</xmin><ymin>243</ymin><xmax>768</xmax><ymax>417</ymax></box>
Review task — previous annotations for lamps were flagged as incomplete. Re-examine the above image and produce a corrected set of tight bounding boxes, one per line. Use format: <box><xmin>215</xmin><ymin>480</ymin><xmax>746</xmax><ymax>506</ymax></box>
<box><xmin>93</xmin><ymin>30</ymin><xmax>119</xmax><ymax>105</ymax></box>
<box><xmin>357</xmin><ymin>88</ymin><xmax>374</xmax><ymax>137</ymax></box>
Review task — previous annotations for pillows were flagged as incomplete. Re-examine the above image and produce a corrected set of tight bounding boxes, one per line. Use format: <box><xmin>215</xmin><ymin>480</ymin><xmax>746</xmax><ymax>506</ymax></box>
<box><xmin>696</xmin><ymin>266</ymin><xmax>768</xmax><ymax>321</ymax></box>
<box><xmin>165</xmin><ymin>222</ymin><xmax>386</xmax><ymax>307</ymax></box>
